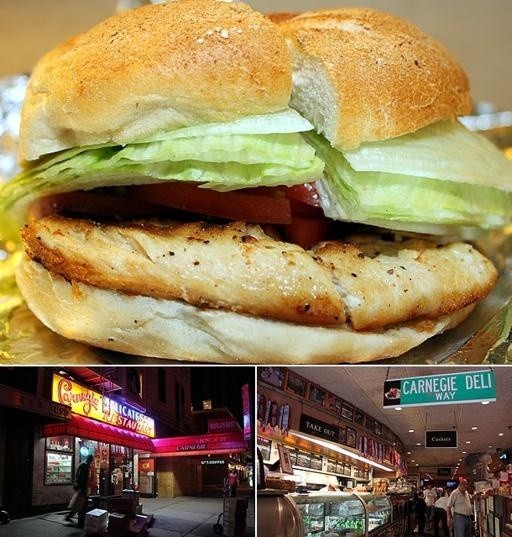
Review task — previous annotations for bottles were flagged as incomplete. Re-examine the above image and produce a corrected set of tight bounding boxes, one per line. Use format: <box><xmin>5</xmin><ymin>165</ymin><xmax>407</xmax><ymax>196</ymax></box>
<box><xmin>333</xmin><ymin>517</ymin><xmax>364</xmax><ymax>537</ymax></box>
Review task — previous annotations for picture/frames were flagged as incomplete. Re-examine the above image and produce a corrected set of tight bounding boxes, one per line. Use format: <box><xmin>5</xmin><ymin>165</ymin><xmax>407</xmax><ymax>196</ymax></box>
<box><xmin>256</xmin><ymin>391</ymin><xmax>290</xmax><ymax>430</ymax></box>
<box><xmin>277</xmin><ymin>443</ymin><xmax>294</xmax><ymax>475</ymax></box>
<box><xmin>358</xmin><ymin>436</ymin><xmax>408</xmax><ymax>472</ymax></box>
<box><xmin>346</xmin><ymin>425</ymin><xmax>356</xmax><ymax>449</ymax></box>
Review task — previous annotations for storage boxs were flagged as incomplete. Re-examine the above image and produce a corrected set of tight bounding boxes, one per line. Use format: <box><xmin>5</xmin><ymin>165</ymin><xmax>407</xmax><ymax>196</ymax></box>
<box><xmin>85</xmin><ymin>509</ymin><xmax>107</xmax><ymax>534</ymax></box>
<box><xmin>108</xmin><ymin>512</ymin><xmax>125</xmax><ymax>529</ymax></box>
<box><xmin>222</xmin><ymin>499</ymin><xmax>247</xmax><ymax>537</ymax></box>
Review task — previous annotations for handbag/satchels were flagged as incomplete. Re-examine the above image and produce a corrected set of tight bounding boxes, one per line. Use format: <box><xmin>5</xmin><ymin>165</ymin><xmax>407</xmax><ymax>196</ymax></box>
<box><xmin>68</xmin><ymin>491</ymin><xmax>86</xmax><ymax>513</ymax></box>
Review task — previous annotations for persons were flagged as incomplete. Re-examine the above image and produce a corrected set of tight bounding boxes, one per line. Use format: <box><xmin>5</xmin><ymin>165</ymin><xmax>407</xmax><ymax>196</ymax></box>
<box><xmin>441</xmin><ymin>491</ymin><xmax>449</xmax><ymax>498</ymax></box>
<box><xmin>432</xmin><ymin>496</ymin><xmax>450</xmax><ymax>537</ymax></box>
<box><xmin>320</xmin><ymin>476</ymin><xmax>340</xmax><ymax>492</ymax></box>
<box><xmin>412</xmin><ymin>492</ymin><xmax>426</xmax><ymax>535</ymax></box>
<box><xmin>448</xmin><ymin>478</ymin><xmax>484</xmax><ymax>537</ymax></box>
<box><xmin>422</xmin><ymin>484</ymin><xmax>438</xmax><ymax>529</ymax></box>
<box><xmin>63</xmin><ymin>454</ymin><xmax>95</xmax><ymax>526</ymax></box>
<box><xmin>227</xmin><ymin>469</ymin><xmax>237</xmax><ymax>496</ymax></box>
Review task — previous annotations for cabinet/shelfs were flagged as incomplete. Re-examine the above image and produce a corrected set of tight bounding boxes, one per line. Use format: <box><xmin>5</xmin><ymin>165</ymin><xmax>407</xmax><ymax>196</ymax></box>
<box><xmin>46</xmin><ymin>459</ymin><xmax>71</xmax><ymax>472</ymax></box>
<box><xmin>257</xmin><ymin>433</ymin><xmax>371</xmax><ymax>488</ymax></box>
<box><xmin>257</xmin><ymin>494</ymin><xmax>393</xmax><ymax>537</ymax></box>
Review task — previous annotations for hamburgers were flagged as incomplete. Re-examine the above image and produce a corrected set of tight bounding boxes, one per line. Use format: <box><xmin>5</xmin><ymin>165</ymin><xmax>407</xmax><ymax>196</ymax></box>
<box><xmin>0</xmin><ymin>2</ymin><xmax>510</xmax><ymax>366</ymax></box>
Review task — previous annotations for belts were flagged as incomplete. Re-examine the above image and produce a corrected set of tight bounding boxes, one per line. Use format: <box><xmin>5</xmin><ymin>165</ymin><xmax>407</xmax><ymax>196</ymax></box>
<box><xmin>454</xmin><ymin>512</ymin><xmax>471</xmax><ymax>518</ymax></box>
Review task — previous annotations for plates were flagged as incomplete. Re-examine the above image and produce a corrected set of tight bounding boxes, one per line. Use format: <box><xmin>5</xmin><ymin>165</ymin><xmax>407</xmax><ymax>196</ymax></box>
<box><xmin>354</xmin><ymin>100</ymin><xmax>512</xmax><ymax>362</ymax></box>
